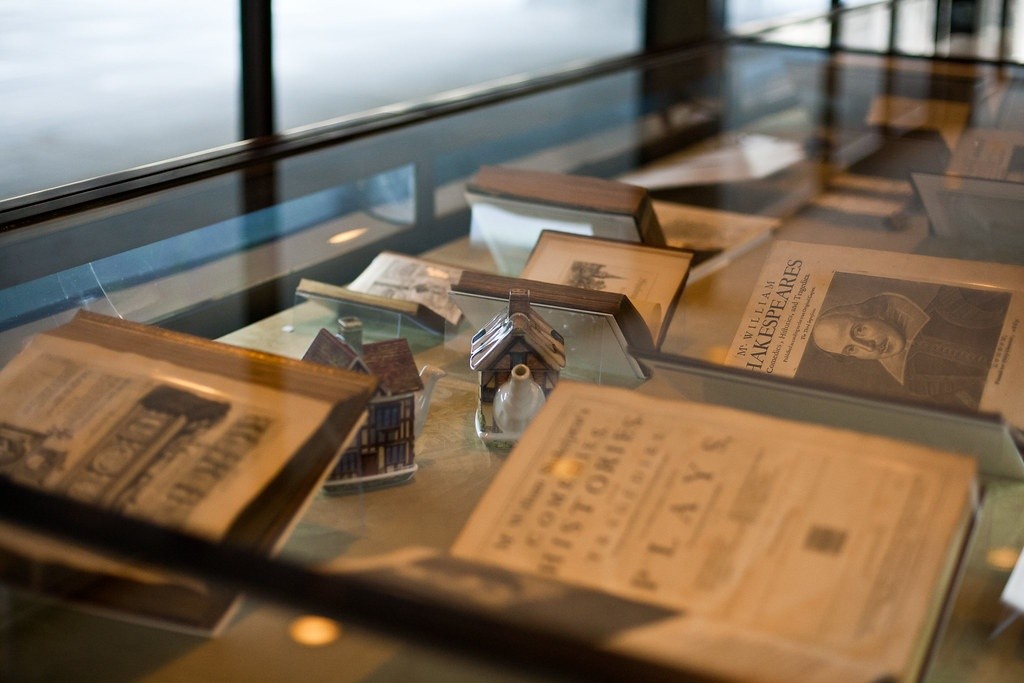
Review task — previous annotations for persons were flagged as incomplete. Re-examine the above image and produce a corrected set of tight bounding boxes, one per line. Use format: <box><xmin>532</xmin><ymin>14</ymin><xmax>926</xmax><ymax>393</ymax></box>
<box><xmin>814</xmin><ymin>285</ymin><xmax>1012</xmax><ymax>410</ymax></box>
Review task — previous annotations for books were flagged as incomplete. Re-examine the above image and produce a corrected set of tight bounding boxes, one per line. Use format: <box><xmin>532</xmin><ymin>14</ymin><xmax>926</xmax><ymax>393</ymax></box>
<box><xmin>296</xmin><ymin>250</ymin><xmax>474</xmax><ymax>341</ymax></box>
<box><xmin>0</xmin><ymin>307</ymin><xmax>379</xmax><ymax>638</ymax></box>
<box><xmin>638</xmin><ymin>239</ymin><xmax>1023</xmax><ymax>477</ymax></box>
<box><xmin>448</xmin><ymin>229</ymin><xmax>692</xmax><ymax>351</ymax></box>
<box><xmin>334</xmin><ymin>379</ymin><xmax>985</xmax><ymax>683</ymax></box>
<box><xmin>466</xmin><ymin>162</ymin><xmax>781</xmax><ymax>262</ymax></box>
